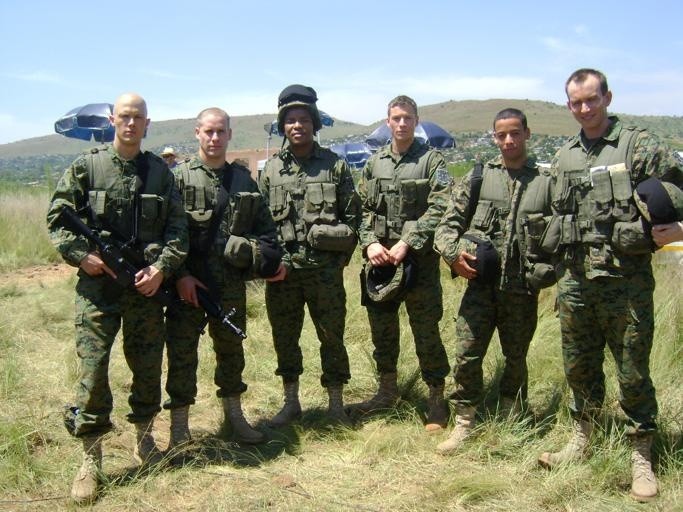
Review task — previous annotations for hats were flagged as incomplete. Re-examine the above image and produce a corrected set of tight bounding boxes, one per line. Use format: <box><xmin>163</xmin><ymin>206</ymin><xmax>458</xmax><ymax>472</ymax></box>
<box><xmin>160</xmin><ymin>147</ymin><xmax>176</xmax><ymax>157</ymax></box>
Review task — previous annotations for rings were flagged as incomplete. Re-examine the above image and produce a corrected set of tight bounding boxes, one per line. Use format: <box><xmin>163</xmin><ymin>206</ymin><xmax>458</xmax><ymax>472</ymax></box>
<box><xmin>179</xmin><ymin>295</ymin><xmax>185</xmax><ymax>301</ymax></box>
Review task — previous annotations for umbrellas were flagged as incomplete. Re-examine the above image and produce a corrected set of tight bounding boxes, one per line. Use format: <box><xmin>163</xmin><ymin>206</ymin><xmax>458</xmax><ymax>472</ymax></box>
<box><xmin>266</xmin><ymin>107</ymin><xmax>336</xmax><ymax>136</ymax></box>
<box><xmin>50</xmin><ymin>101</ymin><xmax>147</xmax><ymax>147</ymax></box>
<box><xmin>327</xmin><ymin>142</ymin><xmax>370</xmax><ymax>168</ymax></box>
<box><xmin>362</xmin><ymin>119</ymin><xmax>457</xmax><ymax>149</ymax></box>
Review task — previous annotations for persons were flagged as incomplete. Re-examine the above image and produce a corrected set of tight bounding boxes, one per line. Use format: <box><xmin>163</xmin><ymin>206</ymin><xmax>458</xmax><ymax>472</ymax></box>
<box><xmin>533</xmin><ymin>67</ymin><xmax>683</xmax><ymax>503</ymax></box>
<box><xmin>431</xmin><ymin>106</ymin><xmax>552</xmax><ymax>456</ymax></box>
<box><xmin>356</xmin><ymin>92</ymin><xmax>454</xmax><ymax>433</ymax></box>
<box><xmin>256</xmin><ymin>84</ymin><xmax>362</xmax><ymax>426</ymax></box>
<box><xmin>155</xmin><ymin>139</ymin><xmax>178</xmax><ymax>167</ymax></box>
<box><xmin>45</xmin><ymin>91</ymin><xmax>191</xmax><ymax>505</ymax></box>
<box><xmin>162</xmin><ymin>105</ymin><xmax>288</xmax><ymax>466</ymax></box>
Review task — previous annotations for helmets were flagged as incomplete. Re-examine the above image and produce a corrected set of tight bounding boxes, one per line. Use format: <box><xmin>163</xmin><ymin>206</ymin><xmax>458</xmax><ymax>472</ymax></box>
<box><xmin>242</xmin><ymin>233</ymin><xmax>281</xmax><ymax>281</ymax></box>
<box><xmin>454</xmin><ymin>229</ymin><xmax>499</xmax><ymax>289</ymax></box>
<box><xmin>277</xmin><ymin>84</ymin><xmax>323</xmax><ymax>135</ymax></box>
<box><xmin>366</xmin><ymin>249</ymin><xmax>421</xmax><ymax>302</ymax></box>
<box><xmin>633</xmin><ymin>179</ymin><xmax>682</xmax><ymax>225</ymax></box>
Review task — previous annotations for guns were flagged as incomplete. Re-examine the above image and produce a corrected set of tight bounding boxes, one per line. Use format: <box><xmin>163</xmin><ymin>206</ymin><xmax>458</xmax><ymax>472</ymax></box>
<box><xmin>59</xmin><ymin>206</ymin><xmax>246</xmax><ymax>338</ymax></box>
